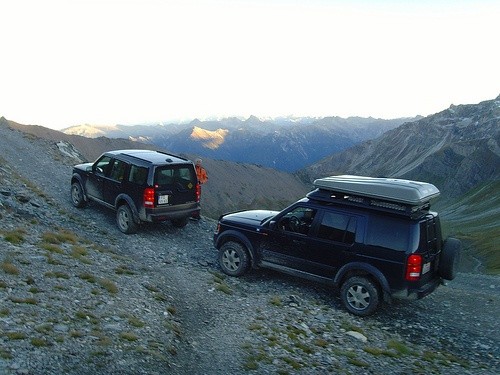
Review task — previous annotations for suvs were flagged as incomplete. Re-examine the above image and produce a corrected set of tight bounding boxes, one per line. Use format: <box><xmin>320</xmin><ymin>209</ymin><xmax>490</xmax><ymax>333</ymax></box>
<box><xmin>212</xmin><ymin>174</ymin><xmax>462</xmax><ymax>317</ymax></box>
<box><xmin>70</xmin><ymin>149</ymin><xmax>201</xmax><ymax>235</ymax></box>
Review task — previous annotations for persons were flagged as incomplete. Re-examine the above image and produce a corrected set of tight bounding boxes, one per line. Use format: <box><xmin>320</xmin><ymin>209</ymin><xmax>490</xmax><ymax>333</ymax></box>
<box><xmin>189</xmin><ymin>159</ymin><xmax>209</xmax><ymax>220</ymax></box>
